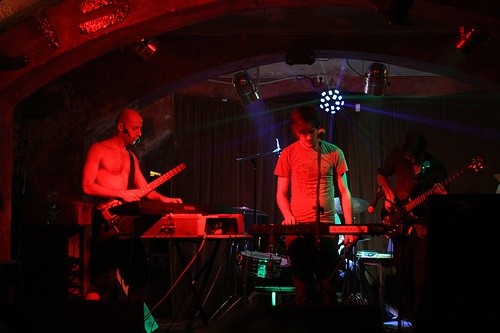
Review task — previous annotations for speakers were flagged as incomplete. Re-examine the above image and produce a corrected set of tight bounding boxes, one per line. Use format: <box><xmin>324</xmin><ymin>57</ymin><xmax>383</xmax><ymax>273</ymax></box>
<box><xmin>424</xmin><ymin>193</ymin><xmax>500</xmax><ymax>333</ymax></box>
<box><xmin>196</xmin><ymin>303</ymin><xmax>381</xmax><ymax>333</ymax></box>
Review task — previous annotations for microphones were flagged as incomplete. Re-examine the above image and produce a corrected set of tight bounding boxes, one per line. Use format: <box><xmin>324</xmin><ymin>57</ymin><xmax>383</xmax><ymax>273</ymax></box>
<box><xmin>367</xmin><ymin>185</ymin><xmax>382</xmax><ymax>213</ymax></box>
<box><xmin>276</xmin><ymin>139</ymin><xmax>282</xmax><ymax>156</ymax></box>
<box><xmin>318</xmin><ymin>128</ymin><xmax>327</xmax><ymax>140</ymax></box>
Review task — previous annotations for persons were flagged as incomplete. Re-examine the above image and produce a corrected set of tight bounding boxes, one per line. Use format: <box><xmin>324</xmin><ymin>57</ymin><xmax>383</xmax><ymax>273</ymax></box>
<box><xmin>80</xmin><ymin>108</ymin><xmax>183</xmax><ymax>301</ymax></box>
<box><xmin>273</xmin><ymin>105</ymin><xmax>359</xmax><ymax>305</ymax></box>
<box><xmin>376</xmin><ymin>130</ymin><xmax>448</xmax><ymax>310</ymax></box>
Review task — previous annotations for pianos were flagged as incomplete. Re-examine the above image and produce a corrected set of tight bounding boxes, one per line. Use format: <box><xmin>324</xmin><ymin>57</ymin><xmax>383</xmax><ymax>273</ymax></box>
<box><xmin>247</xmin><ymin>221</ymin><xmax>389</xmax><ymax>303</ymax></box>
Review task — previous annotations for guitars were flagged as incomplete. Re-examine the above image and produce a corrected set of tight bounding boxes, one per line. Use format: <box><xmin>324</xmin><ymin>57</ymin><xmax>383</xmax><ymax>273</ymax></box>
<box><xmin>380</xmin><ymin>154</ymin><xmax>486</xmax><ymax>242</ymax></box>
<box><xmin>93</xmin><ymin>163</ymin><xmax>187</xmax><ymax>229</ymax></box>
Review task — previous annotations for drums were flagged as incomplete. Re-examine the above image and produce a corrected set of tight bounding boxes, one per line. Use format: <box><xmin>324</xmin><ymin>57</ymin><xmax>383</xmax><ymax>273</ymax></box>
<box><xmin>235</xmin><ymin>254</ymin><xmax>282</xmax><ymax>279</ymax></box>
<box><xmin>236</xmin><ymin>231</ymin><xmax>286</xmax><ymax>260</ymax></box>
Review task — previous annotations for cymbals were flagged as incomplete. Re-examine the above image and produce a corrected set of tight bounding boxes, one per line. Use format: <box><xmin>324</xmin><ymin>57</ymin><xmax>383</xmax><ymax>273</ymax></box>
<box><xmin>333</xmin><ymin>197</ymin><xmax>371</xmax><ymax>215</ymax></box>
<box><xmin>232</xmin><ymin>205</ymin><xmax>262</xmax><ymax>213</ymax></box>
